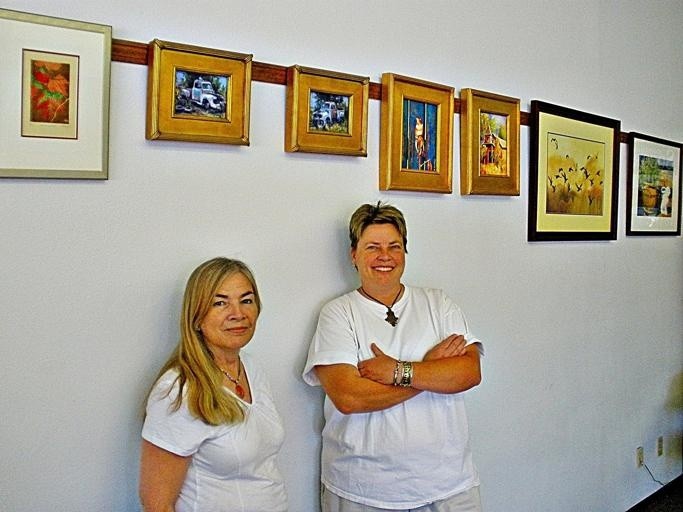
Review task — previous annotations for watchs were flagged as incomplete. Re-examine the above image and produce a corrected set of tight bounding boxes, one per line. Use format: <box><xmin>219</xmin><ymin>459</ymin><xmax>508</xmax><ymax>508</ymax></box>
<box><xmin>400</xmin><ymin>361</ymin><xmax>412</xmax><ymax>389</ymax></box>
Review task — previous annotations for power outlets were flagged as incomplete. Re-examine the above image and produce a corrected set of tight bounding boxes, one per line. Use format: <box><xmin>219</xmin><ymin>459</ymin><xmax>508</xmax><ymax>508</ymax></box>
<box><xmin>637</xmin><ymin>446</ymin><xmax>646</xmax><ymax>468</ymax></box>
<box><xmin>657</xmin><ymin>435</ymin><xmax>663</xmax><ymax>457</ymax></box>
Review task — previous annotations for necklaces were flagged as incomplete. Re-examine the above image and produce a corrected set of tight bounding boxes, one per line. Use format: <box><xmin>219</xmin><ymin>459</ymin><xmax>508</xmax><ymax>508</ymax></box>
<box><xmin>362</xmin><ymin>283</ymin><xmax>404</xmax><ymax>328</ymax></box>
<box><xmin>206</xmin><ymin>356</ymin><xmax>245</xmax><ymax>399</ymax></box>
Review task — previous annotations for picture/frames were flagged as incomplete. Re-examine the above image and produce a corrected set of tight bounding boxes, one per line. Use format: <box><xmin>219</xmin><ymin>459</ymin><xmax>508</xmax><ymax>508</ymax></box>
<box><xmin>460</xmin><ymin>88</ymin><xmax>520</xmax><ymax>196</ymax></box>
<box><xmin>380</xmin><ymin>73</ymin><xmax>455</xmax><ymax>193</ymax></box>
<box><xmin>527</xmin><ymin>100</ymin><xmax>621</xmax><ymax>242</ymax></box>
<box><xmin>284</xmin><ymin>64</ymin><xmax>370</xmax><ymax>158</ymax></box>
<box><xmin>0</xmin><ymin>9</ymin><xmax>112</xmax><ymax>180</ymax></box>
<box><xmin>626</xmin><ymin>132</ymin><xmax>683</xmax><ymax>236</ymax></box>
<box><xmin>146</xmin><ymin>39</ymin><xmax>253</xmax><ymax>147</ymax></box>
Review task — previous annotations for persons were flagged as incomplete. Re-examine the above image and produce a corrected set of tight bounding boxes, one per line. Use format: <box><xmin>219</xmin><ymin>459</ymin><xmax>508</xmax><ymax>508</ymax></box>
<box><xmin>414</xmin><ymin>117</ymin><xmax>429</xmax><ymax>170</ymax></box>
<box><xmin>302</xmin><ymin>200</ymin><xmax>487</xmax><ymax>512</ymax></box>
<box><xmin>137</xmin><ymin>257</ymin><xmax>287</xmax><ymax>511</ymax></box>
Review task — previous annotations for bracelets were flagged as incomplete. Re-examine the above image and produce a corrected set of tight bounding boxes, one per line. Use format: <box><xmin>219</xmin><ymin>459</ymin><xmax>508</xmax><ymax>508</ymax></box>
<box><xmin>393</xmin><ymin>360</ymin><xmax>400</xmax><ymax>388</ymax></box>
